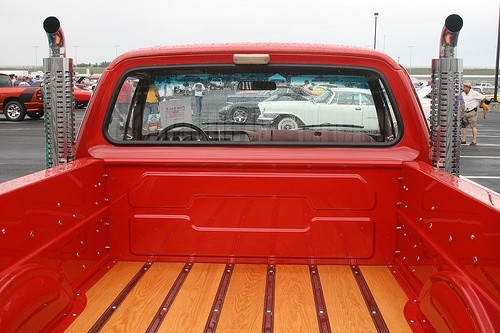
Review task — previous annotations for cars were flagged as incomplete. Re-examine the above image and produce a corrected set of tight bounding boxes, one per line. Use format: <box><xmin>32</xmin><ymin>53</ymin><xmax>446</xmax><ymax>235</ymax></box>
<box><xmin>71</xmin><ymin>77</ymin><xmax>191</xmax><ymax>109</ymax></box>
<box><xmin>218</xmin><ymin>93</ymin><xmax>308</xmax><ymax>124</ymax></box>
<box><xmin>473</xmin><ymin>83</ymin><xmax>496</xmax><ymax>102</ymax></box>
<box><xmin>224</xmin><ymin>84</ymin><xmax>310</xmax><ymax>105</ymax></box>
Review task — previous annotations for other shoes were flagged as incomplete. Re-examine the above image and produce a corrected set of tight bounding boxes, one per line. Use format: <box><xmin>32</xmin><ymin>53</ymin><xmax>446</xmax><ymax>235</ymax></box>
<box><xmin>461</xmin><ymin>140</ymin><xmax>466</xmax><ymax>144</ymax></box>
<box><xmin>128</xmin><ymin>127</ymin><xmax>131</xmax><ymax>130</ymax></box>
<box><xmin>470</xmin><ymin>142</ymin><xmax>477</xmax><ymax>145</ymax></box>
<box><xmin>120</xmin><ymin>125</ymin><xmax>124</xmax><ymax>130</ymax></box>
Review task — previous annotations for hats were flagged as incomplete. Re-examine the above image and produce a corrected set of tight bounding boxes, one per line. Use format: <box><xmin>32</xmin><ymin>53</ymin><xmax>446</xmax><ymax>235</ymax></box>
<box><xmin>463</xmin><ymin>83</ymin><xmax>471</xmax><ymax>89</ymax></box>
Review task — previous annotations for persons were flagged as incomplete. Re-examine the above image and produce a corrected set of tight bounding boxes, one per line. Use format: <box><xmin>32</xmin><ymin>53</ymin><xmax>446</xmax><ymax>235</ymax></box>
<box><xmin>72</xmin><ymin>76</ymin><xmax>95</xmax><ymax>86</ymax></box>
<box><xmin>231</xmin><ymin>80</ymin><xmax>239</xmax><ymax>90</ymax></box>
<box><xmin>301</xmin><ymin>80</ymin><xmax>313</xmax><ymax>90</ymax></box>
<box><xmin>142</xmin><ymin>83</ymin><xmax>162</xmax><ymax>131</ymax></box>
<box><xmin>116</xmin><ymin>77</ymin><xmax>133</xmax><ymax>130</ymax></box>
<box><xmin>9</xmin><ymin>74</ymin><xmax>43</xmax><ymax>87</ymax></box>
<box><xmin>165</xmin><ymin>79</ymin><xmax>175</xmax><ymax>100</ymax></box>
<box><xmin>191</xmin><ymin>77</ymin><xmax>205</xmax><ymax>115</ymax></box>
<box><xmin>454</xmin><ymin>92</ymin><xmax>465</xmax><ymax>113</ymax></box>
<box><xmin>426</xmin><ymin>79</ymin><xmax>431</xmax><ymax>86</ymax></box>
<box><xmin>461</xmin><ymin>83</ymin><xmax>486</xmax><ymax>146</ymax></box>
<box><xmin>183</xmin><ymin>78</ymin><xmax>189</xmax><ymax>96</ymax></box>
<box><xmin>156</xmin><ymin>75</ymin><xmax>166</xmax><ymax>101</ymax></box>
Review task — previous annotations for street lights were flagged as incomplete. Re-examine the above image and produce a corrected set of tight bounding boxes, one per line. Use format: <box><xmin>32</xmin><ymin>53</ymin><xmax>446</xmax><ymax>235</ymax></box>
<box><xmin>373</xmin><ymin>12</ymin><xmax>380</xmax><ymax>49</ymax></box>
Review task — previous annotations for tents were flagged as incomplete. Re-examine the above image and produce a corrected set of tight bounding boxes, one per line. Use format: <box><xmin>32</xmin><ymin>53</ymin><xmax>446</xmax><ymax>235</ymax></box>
<box><xmin>268</xmin><ymin>73</ymin><xmax>286</xmax><ymax>82</ymax></box>
<box><xmin>312</xmin><ymin>75</ymin><xmax>329</xmax><ymax>81</ymax></box>
<box><xmin>357</xmin><ymin>82</ymin><xmax>369</xmax><ymax>89</ymax></box>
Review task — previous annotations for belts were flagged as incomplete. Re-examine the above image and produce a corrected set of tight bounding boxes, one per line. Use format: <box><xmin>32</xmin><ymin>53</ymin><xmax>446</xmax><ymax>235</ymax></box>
<box><xmin>464</xmin><ymin>107</ymin><xmax>477</xmax><ymax>113</ymax></box>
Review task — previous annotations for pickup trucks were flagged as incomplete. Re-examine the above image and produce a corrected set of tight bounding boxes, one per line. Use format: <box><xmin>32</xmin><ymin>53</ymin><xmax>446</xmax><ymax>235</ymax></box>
<box><xmin>0</xmin><ymin>13</ymin><xmax>500</xmax><ymax>332</ymax></box>
<box><xmin>0</xmin><ymin>73</ymin><xmax>44</xmax><ymax>120</ymax></box>
<box><xmin>256</xmin><ymin>87</ymin><xmax>432</xmax><ymax>130</ymax></box>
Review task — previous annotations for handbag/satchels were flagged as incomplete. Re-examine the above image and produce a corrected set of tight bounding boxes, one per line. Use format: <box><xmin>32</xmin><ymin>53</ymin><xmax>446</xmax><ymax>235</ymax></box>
<box><xmin>202</xmin><ymin>92</ymin><xmax>205</xmax><ymax>95</ymax></box>
